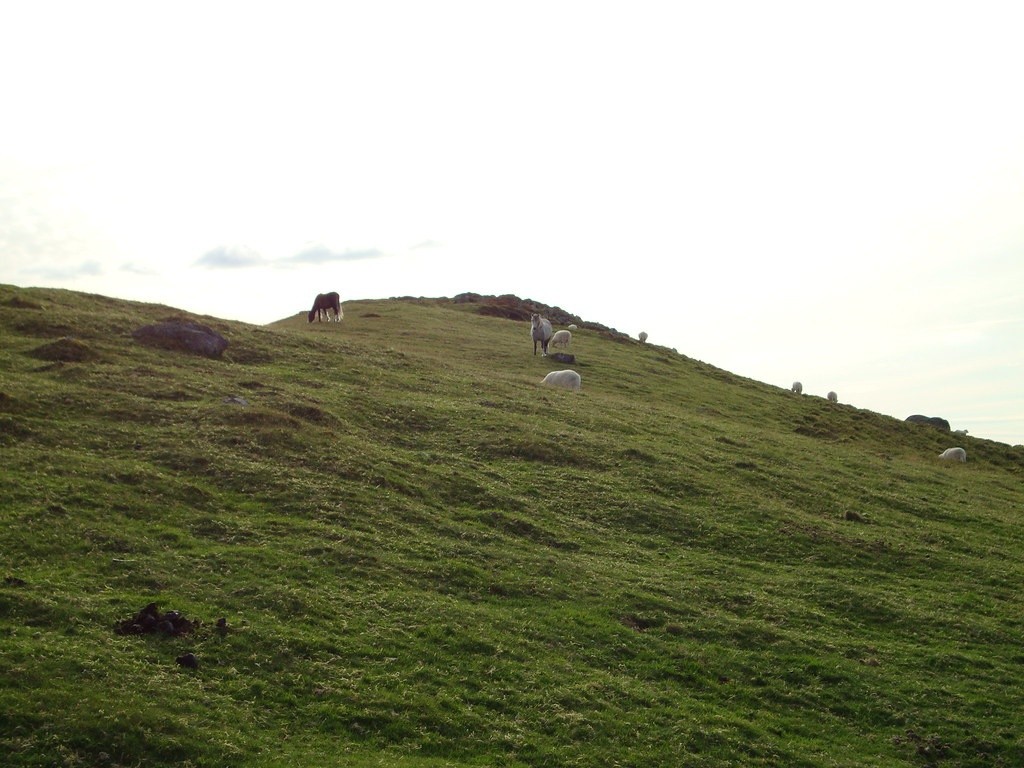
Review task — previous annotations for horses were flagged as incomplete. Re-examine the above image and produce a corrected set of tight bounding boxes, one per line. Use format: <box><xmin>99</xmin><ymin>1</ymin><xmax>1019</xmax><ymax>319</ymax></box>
<box><xmin>308</xmin><ymin>292</ymin><xmax>343</xmax><ymax>323</ymax></box>
<box><xmin>530</xmin><ymin>313</ymin><xmax>553</xmax><ymax>357</ymax></box>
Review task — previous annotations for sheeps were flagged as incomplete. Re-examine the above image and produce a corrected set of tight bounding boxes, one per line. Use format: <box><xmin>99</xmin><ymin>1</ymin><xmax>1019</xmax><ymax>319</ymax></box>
<box><xmin>791</xmin><ymin>382</ymin><xmax>802</xmax><ymax>395</ymax></box>
<box><xmin>551</xmin><ymin>324</ymin><xmax>577</xmax><ymax>348</ymax></box>
<box><xmin>639</xmin><ymin>332</ymin><xmax>648</xmax><ymax>344</ymax></box>
<box><xmin>541</xmin><ymin>370</ymin><xmax>581</xmax><ymax>393</ymax></box>
<box><xmin>939</xmin><ymin>429</ymin><xmax>969</xmax><ymax>462</ymax></box>
<box><xmin>827</xmin><ymin>391</ymin><xmax>838</xmax><ymax>403</ymax></box>
